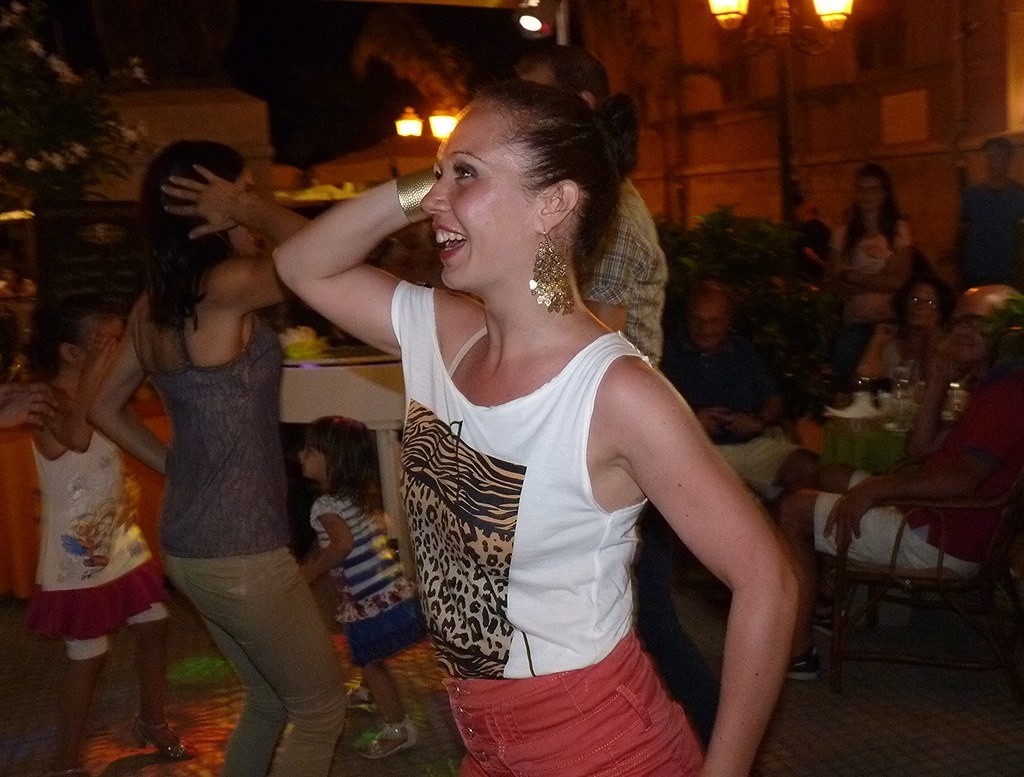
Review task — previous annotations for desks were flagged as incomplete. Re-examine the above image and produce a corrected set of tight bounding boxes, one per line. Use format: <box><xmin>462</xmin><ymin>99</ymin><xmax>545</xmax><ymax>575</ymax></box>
<box><xmin>277</xmin><ymin>343</ymin><xmax>413</xmax><ymax>580</ymax></box>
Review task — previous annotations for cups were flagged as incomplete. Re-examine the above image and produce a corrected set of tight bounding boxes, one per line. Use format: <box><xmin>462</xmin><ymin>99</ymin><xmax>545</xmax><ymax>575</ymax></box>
<box><xmin>851</xmin><ymin>392</ymin><xmax>877</xmax><ymax>411</ymax></box>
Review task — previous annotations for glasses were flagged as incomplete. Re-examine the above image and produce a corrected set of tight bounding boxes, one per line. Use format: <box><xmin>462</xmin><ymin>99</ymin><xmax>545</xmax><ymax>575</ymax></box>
<box><xmin>945</xmin><ymin>315</ymin><xmax>995</xmax><ymax>331</ymax></box>
<box><xmin>687</xmin><ymin>313</ymin><xmax>730</xmax><ymax>330</ymax></box>
<box><xmin>905</xmin><ymin>295</ymin><xmax>939</xmax><ymax>311</ymax></box>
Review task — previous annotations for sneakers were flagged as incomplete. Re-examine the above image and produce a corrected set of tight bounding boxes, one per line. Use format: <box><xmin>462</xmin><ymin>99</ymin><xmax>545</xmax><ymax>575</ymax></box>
<box><xmin>811</xmin><ymin>611</ymin><xmax>834</xmax><ymax>640</ymax></box>
<box><xmin>785</xmin><ymin>644</ymin><xmax>821</xmax><ymax>680</ymax></box>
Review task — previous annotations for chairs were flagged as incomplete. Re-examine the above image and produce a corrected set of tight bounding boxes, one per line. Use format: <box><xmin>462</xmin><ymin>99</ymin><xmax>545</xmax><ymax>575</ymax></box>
<box><xmin>829</xmin><ymin>470</ymin><xmax>1024</xmax><ymax>704</ymax></box>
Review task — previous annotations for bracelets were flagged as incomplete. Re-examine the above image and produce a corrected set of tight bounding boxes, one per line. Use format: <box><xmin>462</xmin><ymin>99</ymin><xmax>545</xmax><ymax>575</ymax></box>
<box><xmin>395</xmin><ymin>165</ymin><xmax>439</xmax><ymax>225</ymax></box>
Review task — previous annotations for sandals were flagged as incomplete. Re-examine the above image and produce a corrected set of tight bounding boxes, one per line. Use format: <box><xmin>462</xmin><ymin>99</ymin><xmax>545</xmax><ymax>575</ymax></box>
<box><xmin>359</xmin><ymin>714</ymin><xmax>417</xmax><ymax>759</ymax></box>
<box><xmin>131</xmin><ymin>716</ymin><xmax>195</xmax><ymax>760</ymax></box>
<box><xmin>346</xmin><ymin>686</ymin><xmax>377</xmax><ymax>712</ymax></box>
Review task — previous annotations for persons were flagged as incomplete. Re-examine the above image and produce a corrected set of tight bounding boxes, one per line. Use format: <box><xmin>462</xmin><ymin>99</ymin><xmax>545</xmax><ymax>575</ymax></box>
<box><xmin>296</xmin><ymin>413</ymin><xmax>431</xmax><ymax>762</ymax></box>
<box><xmin>0</xmin><ymin>134</ymin><xmax>1023</xmax><ymax>508</ymax></box>
<box><xmin>770</xmin><ymin>284</ymin><xmax>1024</xmax><ymax>682</ymax></box>
<box><xmin>502</xmin><ymin>40</ymin><xmax>721</xmax><ymax>755</ymax></box>
<box><xmin>81</xmin><ymin>137</ymin><xmax>348</xmax><ymax>777</ymax></box>
<box><xmin>27</xmin><ymin>297</ymin><xmax>199</xmax><ymax>777</ymax></box>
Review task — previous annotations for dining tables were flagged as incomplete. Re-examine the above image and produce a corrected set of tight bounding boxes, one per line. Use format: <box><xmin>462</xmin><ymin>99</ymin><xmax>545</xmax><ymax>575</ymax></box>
<box><xmin>825</xmin><ymin>412</ymin><xmax>919</xmax><ymax>478</ymax></box>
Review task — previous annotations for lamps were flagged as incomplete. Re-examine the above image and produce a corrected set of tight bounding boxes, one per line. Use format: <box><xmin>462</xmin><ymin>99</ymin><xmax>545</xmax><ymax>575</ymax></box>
<box><xmin>395</xmin><ymin>108</ymin><xmax>460</xmax><ymax>139</ymax></box>
<box><xmin>708</xmin><ymin>0</ymin><xmax>853</xmax><ymax>56</ymax></box>
<box><xmin>514</xmin><ymin>0</ymin><xmax>561</xmax><ymax>39</ymax></box>
